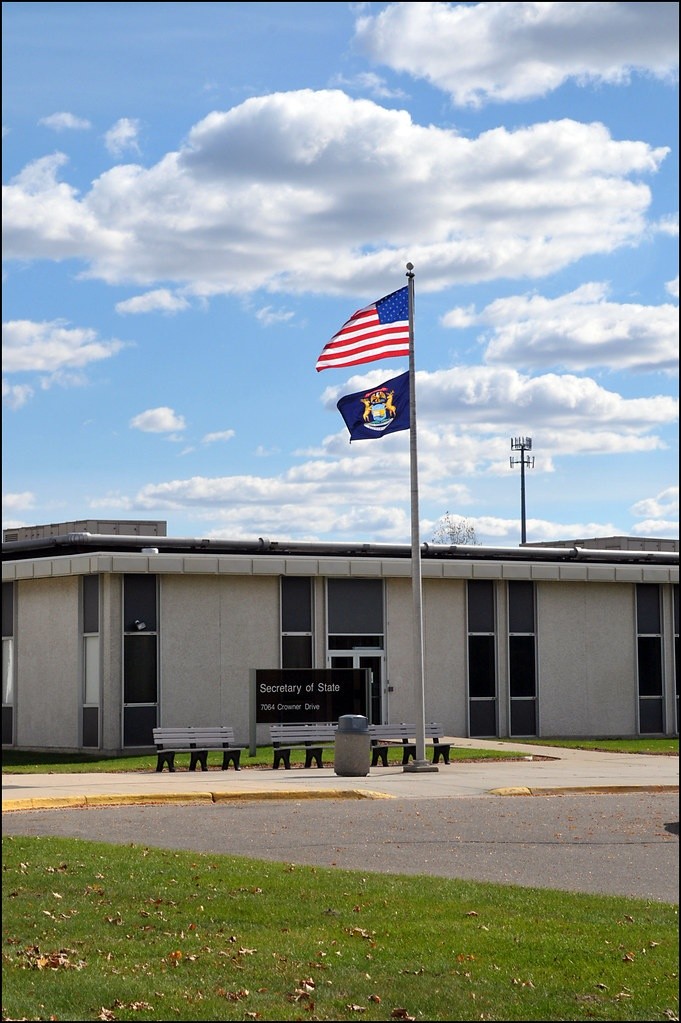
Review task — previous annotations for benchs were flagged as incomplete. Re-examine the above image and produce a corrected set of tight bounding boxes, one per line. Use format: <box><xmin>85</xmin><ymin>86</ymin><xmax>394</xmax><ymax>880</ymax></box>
<box><xmin>369</xmin><ymin>722</ymin><xmax>455</xmax><ymax>766</ymax></box>
<box><xmin>270</xmin><ymin>726</ymin><xmax>337</xmax><ymax>768</ymax></box>
<box><xmin>152</xmin><ymin>727</ymin><xmax>246</xmax><ymax>770</ymax></box>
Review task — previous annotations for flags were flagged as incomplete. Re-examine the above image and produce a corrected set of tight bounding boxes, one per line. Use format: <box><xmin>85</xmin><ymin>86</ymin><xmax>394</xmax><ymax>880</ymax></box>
<box><xmin>336</xmin><ymin>370</ymin><xmax>411</xmax><ymax>444</ymax></box>
<box><xmin>315</xmin><ymin>284</ymin><xmax>410</xmax><ymax>371</ymax></box>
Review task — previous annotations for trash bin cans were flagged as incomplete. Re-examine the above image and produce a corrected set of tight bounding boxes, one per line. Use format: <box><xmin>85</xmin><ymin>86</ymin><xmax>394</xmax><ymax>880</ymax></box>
<box><xmin>333</xmin><ymin>715</ymin><xmax>370</xmax><ymax>776</ymax></box>
